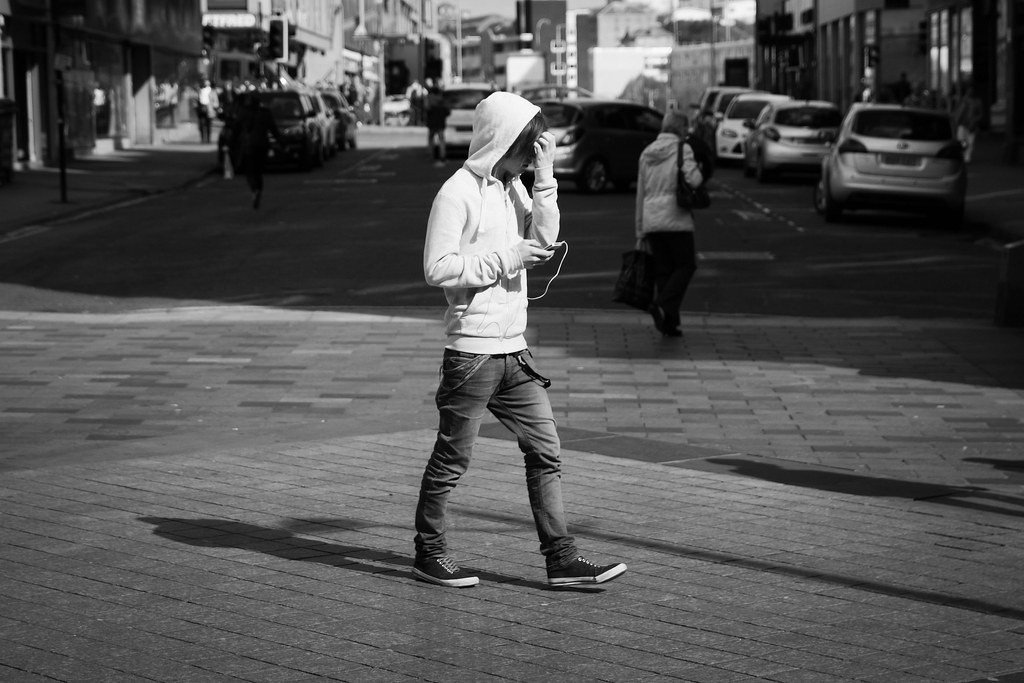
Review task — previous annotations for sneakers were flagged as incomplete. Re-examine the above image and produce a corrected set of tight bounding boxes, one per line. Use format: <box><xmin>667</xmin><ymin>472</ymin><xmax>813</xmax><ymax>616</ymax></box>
<box><xmin>411</xmin><ymin>553</ymin><xmax>480</xmax><ymax>588</ymax></box>
<box><xmin>547</xmin><ymin>555</ymin><xmax>627</xmax><ymax>587</ymax></box>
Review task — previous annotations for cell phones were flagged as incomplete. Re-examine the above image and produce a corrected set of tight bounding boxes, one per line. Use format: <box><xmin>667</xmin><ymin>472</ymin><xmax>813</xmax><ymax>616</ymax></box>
<box><xmin>543</xmin><ymin>243</ymin><xmax>562</xmax><ymax>252</ymax></box>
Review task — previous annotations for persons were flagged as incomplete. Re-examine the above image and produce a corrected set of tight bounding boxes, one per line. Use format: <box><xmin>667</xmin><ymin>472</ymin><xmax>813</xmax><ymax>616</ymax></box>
<box><xmin>689</xmin><ymin>110</ymin><xmax>720</xmax><ymax>210</ymax></box>
<box><xmin>168</xmin><ymin>75</ymin><xmax>379</xmax><ymax>142</ymax></box>
<box><xmin>234</xmin><ymin>97</ymin><xmax>289</xmax><ymax>202</ymax></box>
<box><xmin>891</xmin><ymin>70</ymin><xmax>936</xmax><ymax>109</ymax></box>
<box><xmin>410</xmin><ymin>86</ymin><xmax>450</xmax><ymax>162</ymax></box>
<box><xmin>635</xmin><ymin>110</ymin><xmax>703</xmax><ymax>341</ymax></box>
<box><xmin>411</xmin><ymin>92</ymin><xmax>627</xmax><ymax>590</ymax></box>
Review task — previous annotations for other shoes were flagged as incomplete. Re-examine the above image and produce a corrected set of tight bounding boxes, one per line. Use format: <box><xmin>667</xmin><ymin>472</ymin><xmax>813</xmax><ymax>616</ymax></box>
<box><xmin>661</xmin><ymin>325</ymin><xmax>683</xmax><ymax>337</ymax></box>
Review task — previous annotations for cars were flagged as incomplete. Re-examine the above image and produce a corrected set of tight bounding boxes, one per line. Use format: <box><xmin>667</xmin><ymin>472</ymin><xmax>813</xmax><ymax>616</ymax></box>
<box><xmin>742</xmin><ymin>102</ymin><xmax>842</xmax><ymax>186</ymax></box>
<box><xmin>215</xmin><ymin>87</ymin><xmax>356</xmax><ymax>176</ymax></box>
<box><xmin>714</xmin><ymin>92</ymin><xmax>794</xmax><ymax>165</ymax></box>
<box><xmin>687</xmin><ymin>85</ymin><xmax>774</xmax><ymax>150</ymax></box>
<box><xmin>813</xmin><ymin>102</ymin><xmax>968</xmax><ymax>227</ymax></box>
<box><xmin>514</xmin><ymin>83</ymin><xmax>597</xmax><ymax>104</ymax></box>
<box><xmin>517</xmin><ymin>100</ymin><xmax>716</xmax><ymax>198</ymax></box>
<box><xmin>440</xmin><ymin>81</ymin><xmax>501</xmax><ymax>156</ymax></box>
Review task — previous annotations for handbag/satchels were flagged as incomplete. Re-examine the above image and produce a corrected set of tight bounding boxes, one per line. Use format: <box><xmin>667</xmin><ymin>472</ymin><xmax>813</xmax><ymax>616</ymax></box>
<box><xmin>611</xmin><ymin>239</ymin><xmax>656</xmax><ymax>313</ymax></box>
<box><xmin>677</xmin><ymin>139</ymin><xmax>712</xmax><ymax>211</ymax></box>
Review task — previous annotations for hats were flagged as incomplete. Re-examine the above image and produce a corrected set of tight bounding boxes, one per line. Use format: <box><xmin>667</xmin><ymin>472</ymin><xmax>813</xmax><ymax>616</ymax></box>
<box><xmin>661</xmin><ymin>110</ymin><xmax>689</xmax><ymax>138</ymax></box>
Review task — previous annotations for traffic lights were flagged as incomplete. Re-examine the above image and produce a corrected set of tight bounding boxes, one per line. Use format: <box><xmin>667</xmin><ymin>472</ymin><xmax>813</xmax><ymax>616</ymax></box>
<box><xmin>266</xmin><ymin>17</ymin><xmax>288</xmax><ymax>64</ymax></box>
